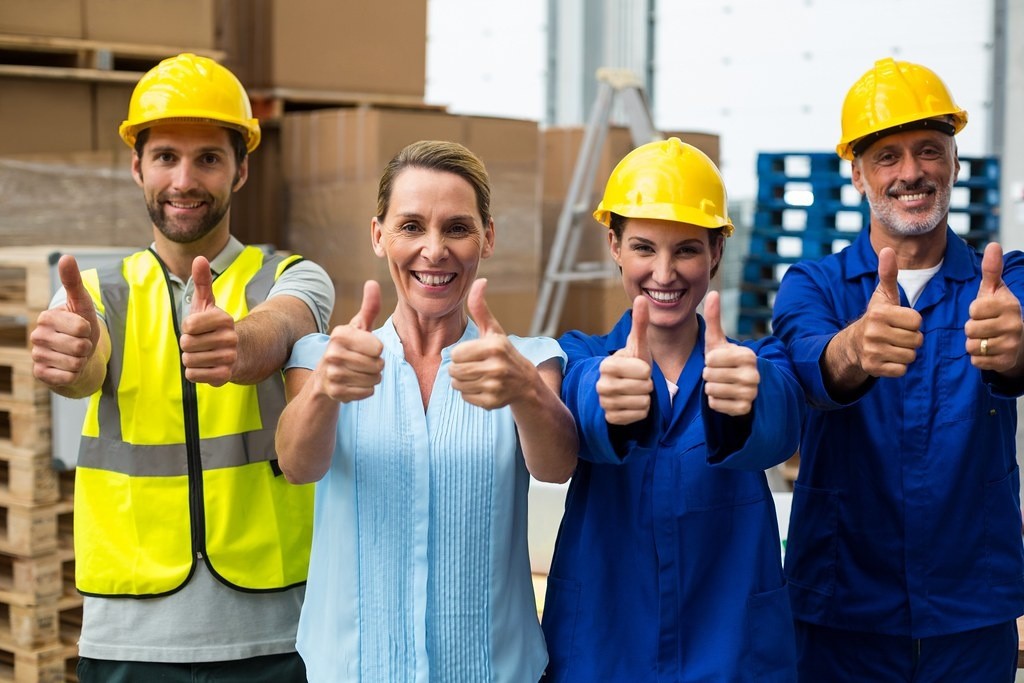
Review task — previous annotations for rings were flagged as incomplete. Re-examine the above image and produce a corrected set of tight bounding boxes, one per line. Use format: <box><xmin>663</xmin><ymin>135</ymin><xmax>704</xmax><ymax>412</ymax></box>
<box><xmin>981</xmin><ymin>338</ymin><xmax>988</xmax><ymax>356</ymax></box>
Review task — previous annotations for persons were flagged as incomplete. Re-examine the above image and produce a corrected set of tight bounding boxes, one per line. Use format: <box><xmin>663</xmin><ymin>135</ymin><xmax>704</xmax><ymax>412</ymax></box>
<box><xmin>774</xmin><ymin>61</ymin><xmax>1024</xmax><ymax>683</ymax></box>
<box><xmin>274</xmin><ymin>142</ymin><xmax>576</xmax><ymax>683</ymax></box>
<box><xmin>29</xmin><ymin>53</ymin><xmax>334</xmax><ymax>683</ymax></box>
<box><xmin>543</xmin><ymin>139</ymin><xmax>803</xmax><ymax>683</ymax></box>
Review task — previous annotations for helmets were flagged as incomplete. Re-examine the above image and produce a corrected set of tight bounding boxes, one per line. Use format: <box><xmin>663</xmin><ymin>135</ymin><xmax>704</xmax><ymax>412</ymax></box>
<box><xmin>836</xmin><ymin>57</ymin><xmax>968</xmax><ymax>161</ymax></box>
<box><xmin>119</xmin><ymin>52</ymin><xmax>262</xmax><ymax>154</ymax></box>
<box><xmin>592</xmin><ymin>137</ymin><xmax>735</xmax><ymax>238</ymax></box>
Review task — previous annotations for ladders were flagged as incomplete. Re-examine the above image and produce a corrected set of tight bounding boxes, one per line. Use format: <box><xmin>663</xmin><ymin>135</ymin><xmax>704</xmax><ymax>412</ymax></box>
<box><xmin>528</xmin><ymin>67</ymin><xmax>709</xmax><ymax>340</ymax></box>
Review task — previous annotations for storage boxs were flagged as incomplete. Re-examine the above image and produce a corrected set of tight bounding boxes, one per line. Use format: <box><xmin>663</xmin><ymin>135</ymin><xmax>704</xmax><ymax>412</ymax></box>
<box><xmin>0</xmin><ymin>0</ymin><xmax>720</xmax><ymax>334</ymax></box>
